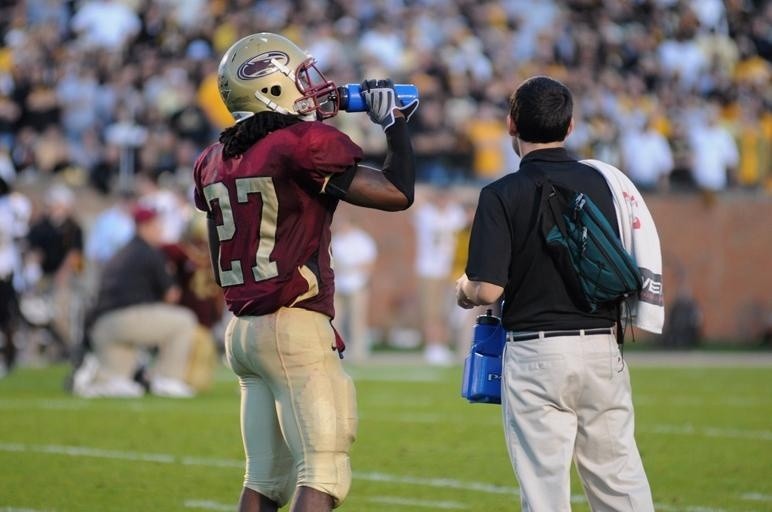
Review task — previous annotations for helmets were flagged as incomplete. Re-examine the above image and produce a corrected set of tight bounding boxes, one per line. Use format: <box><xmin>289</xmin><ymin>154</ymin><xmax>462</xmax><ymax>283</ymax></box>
<box><xmin>216</xmin><ymin>32</ymin><xmax>340</xmax><ymax>122</ymax></box>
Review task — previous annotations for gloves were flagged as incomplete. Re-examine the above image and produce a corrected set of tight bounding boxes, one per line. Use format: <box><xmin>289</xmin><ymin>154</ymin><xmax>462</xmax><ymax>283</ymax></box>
<box><xmin>361</xmin><ymin>78</ymin><xmax>420</xmax><ymax>131</ymax></box>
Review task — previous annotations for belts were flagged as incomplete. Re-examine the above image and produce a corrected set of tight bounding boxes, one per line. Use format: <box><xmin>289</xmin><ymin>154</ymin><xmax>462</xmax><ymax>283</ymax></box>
<box><xmin>508</xmin><ymin>329</ymin><xmax>614</xmax><ymax>342</ymax></box>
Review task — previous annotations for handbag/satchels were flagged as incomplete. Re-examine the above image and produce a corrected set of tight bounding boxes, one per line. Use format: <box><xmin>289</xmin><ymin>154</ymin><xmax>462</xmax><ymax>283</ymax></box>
<box><xmin>540</xmin><ymin>184</ymin><xmax>643</xmax><ymax>309</ymax></box>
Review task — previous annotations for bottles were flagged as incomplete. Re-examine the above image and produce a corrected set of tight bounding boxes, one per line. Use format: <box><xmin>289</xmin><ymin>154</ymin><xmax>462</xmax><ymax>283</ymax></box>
<box><xmin>473</xmin><ymin>309</ymin><xmax>501</xmax><ymax>356</ymax></box>
<box><xmin>335</xmin><ymin>83</ymin><xmax>419</xmax><ymax>113</ymax></box>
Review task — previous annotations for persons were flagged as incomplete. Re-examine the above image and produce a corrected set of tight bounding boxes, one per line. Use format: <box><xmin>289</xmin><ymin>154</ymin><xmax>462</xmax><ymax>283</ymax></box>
<box><xmin>0</xmin><ymin>1</ymin><xmax>772</xmax><ymax>398</ymax></box>
<box><xmin>454</xmin><ymin>75</ymin><xmax>656</xmax><ymax>512</ymax></box>
<box><xmin>192</xmin><ymin>30</ymin><xmax>416</xmax><ymax>512</ymax></box>
